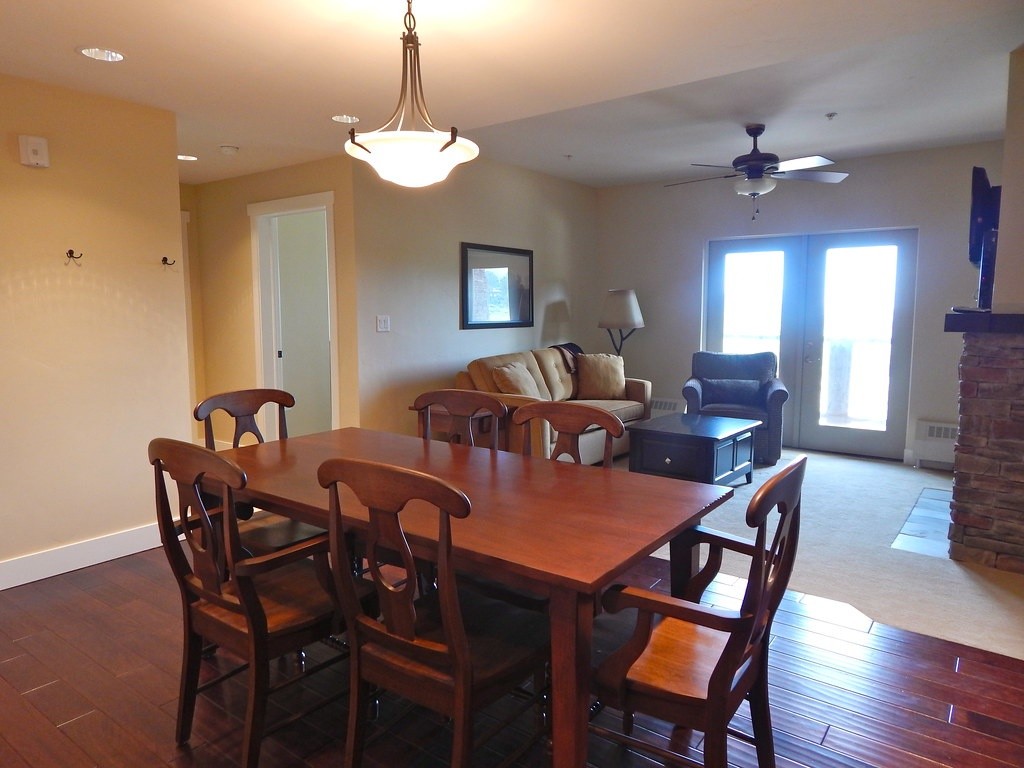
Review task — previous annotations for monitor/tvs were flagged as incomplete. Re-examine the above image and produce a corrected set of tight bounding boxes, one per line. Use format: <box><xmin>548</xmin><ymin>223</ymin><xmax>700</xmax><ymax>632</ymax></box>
<box><xmin>969</xmin><ymin>167</ymin><xmax>990</xmax><ymax>264</ymax></box>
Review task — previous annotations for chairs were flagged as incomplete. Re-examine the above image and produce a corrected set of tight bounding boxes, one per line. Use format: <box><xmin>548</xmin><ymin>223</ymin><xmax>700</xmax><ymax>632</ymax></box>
<box><xmin>433</xmin><ymin>401</ymin><xmax>624</xmax><ymax>655</ymax></box>
<box><xmin>543</xmin><ymin>459</ymin><xmax>805</xmax><ymax>768</ymax></box>
<box><xmin>317</xmin><ymin>458</ymin><xmax>550</xmax><ymax>768</ymax></box>
<box><xmin>149</xmin><ymin>438</ymin><xmax>383</xmax><ymax>768</ymax></box>
<box><xmin>362</xmin><ymin>389</ymin><xmax>508</xmax><ymax>598</ymax></box>
<box><xmin>194</xmin><ymin>389</ymin><xmax>362</xmax><ymax>675</ymax></box>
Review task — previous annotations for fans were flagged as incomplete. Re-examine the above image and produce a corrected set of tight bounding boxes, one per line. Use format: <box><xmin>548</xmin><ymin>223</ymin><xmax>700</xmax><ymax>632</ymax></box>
<box><xmin>664</xmin><ymin>124</ymin><xmax>850</xmax><ymax>221</ymax></box>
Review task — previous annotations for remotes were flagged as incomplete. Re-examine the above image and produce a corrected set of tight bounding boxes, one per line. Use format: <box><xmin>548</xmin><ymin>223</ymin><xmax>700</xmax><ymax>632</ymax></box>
<box><xmin>951</xmin><ymin>306</ymin><xmax>991</xmax><ymax>314</ymax></box>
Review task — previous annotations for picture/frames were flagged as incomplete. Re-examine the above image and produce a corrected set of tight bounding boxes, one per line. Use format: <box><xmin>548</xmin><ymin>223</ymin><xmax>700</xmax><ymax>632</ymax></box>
<box><xmin>461</xmin><ymin>242</ymin><xmax>534</xmax><ymax>329</ymax></box>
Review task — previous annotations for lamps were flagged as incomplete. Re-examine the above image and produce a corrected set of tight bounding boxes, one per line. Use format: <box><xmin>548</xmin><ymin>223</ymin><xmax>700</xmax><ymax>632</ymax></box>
<box><xmin>344</xmin><ymin>0</ymin><xmax>480</xmax><ymax>190</ymax></box>
<box><xmin>597</xmin><ymin>288</ymin><xmax>646</xmax><ymax>356</ymax></box>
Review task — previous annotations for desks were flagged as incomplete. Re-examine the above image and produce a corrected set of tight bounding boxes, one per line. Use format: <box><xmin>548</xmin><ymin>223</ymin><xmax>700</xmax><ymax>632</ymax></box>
<box><xmin>416</xmin><ymin>404</ymin><xmax>518</xmax><ymax>452</ymax></box>
<box><xmin>169</xmin><ymin>426</ymin><xmax>734</xmax><ymax>768</ymax></box>
<box><xmin>624</xmin><ymin>412</ymin><xmax>762</xmax><ymax>485</ymax></box>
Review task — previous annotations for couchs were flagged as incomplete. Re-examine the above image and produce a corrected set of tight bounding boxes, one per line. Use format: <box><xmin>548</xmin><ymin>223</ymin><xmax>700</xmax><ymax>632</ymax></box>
<box><xmin>455</xmin><ymin>343</ymin><xmax>652</xmax><ymax>466</ymax></box>
<box><xmin>682</xmin><ymin>351</ymin><xmax>788</xmax><ymax>466</ymax></box>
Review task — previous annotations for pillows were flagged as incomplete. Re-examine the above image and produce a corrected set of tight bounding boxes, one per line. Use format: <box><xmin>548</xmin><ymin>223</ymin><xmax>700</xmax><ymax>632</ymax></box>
<box><xmin>493</xmin><ymin>362</ymin><xmax>542</xmax><ymax>398</ymax></box>
<box><xmin>576</xmin><ymin>353</ymin><xmax>627</xmax><ymax>400</ymax></box>
